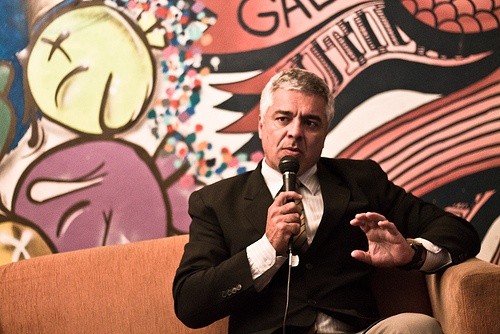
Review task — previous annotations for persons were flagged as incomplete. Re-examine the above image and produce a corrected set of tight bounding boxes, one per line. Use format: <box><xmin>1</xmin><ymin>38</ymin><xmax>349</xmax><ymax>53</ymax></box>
<box><xmin>172</xmin><ymin>68</ymin><xmax>481</xmax><ymax>334</ymax></box>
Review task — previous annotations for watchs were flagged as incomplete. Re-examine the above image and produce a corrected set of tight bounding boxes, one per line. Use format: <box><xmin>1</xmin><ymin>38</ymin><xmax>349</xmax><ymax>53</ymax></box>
<box><xmin>405</xmin><ymin>239</ymin><xmax>424</xmax><ymax>266</ymax></box>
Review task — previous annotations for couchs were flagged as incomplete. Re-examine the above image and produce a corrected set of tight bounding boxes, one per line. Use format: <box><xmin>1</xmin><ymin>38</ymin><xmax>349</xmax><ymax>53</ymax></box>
<box><xmin>0</xmin><ymin>232</ymin><xmax>500</xmax><ymax>334</ymax></box>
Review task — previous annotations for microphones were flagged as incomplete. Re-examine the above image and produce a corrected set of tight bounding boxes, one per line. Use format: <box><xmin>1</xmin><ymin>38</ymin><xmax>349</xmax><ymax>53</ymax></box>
<box><xmin>278</xmin><ymin>155</ymin><xmax>300</xmax><ymax>250</ymax></box>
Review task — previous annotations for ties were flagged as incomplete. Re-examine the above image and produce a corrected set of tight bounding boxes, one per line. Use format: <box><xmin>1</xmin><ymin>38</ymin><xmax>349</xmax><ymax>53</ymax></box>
<box><xmin>293</xmin><ymin>180</ymin><xmax>308</xmax><ymax>250</ymax></box>
